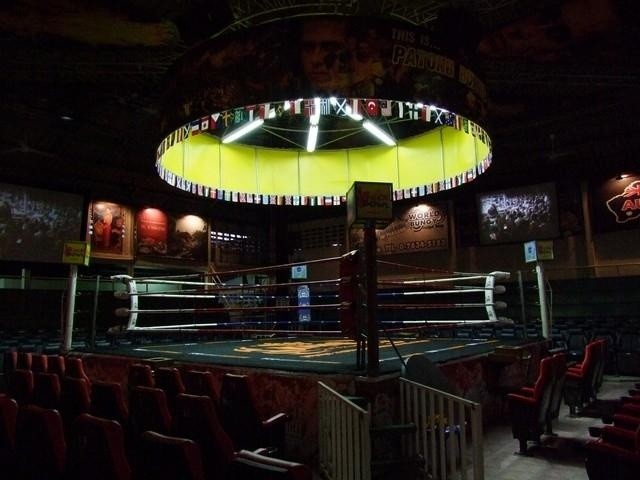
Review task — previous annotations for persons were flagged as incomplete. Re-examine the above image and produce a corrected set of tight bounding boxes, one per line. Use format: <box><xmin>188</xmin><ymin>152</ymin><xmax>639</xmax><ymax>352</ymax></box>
<box><xmin>301</xmin><ymin>19</ymin><xmax>356</xmax><ymax>98</ymax></box>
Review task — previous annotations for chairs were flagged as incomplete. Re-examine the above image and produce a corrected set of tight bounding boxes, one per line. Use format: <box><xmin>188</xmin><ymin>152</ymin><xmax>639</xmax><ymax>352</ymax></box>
<box><xmin>455</xmin><ymin>316</ymin><xmax>639</xmax><ymax>376</ymax></box>
<box><xmin>1</xmin><ymin>349</ymin><xmax>312</xmax><ymax>479</ymax></box>
<box><xmin>509</xmin><ymin>338</ymin><xmax>639</xmax><ymax>480</ymax></box>
<box><xmin>1</xmin><ymin>326</ymin><xmax>206</xmax><ymax>354</ymax></box>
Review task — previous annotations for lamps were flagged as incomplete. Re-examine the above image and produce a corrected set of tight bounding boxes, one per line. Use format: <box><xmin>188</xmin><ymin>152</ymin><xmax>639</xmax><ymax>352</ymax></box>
<box><xmin>363</xmin><ymin>119</ymin><xmax>398</xmax><ymax>146</ymax></box>
<box><xmin>220</xmin><ymin>116</ymin><xmax>265</xmax><ymax>145</ymax></box>
